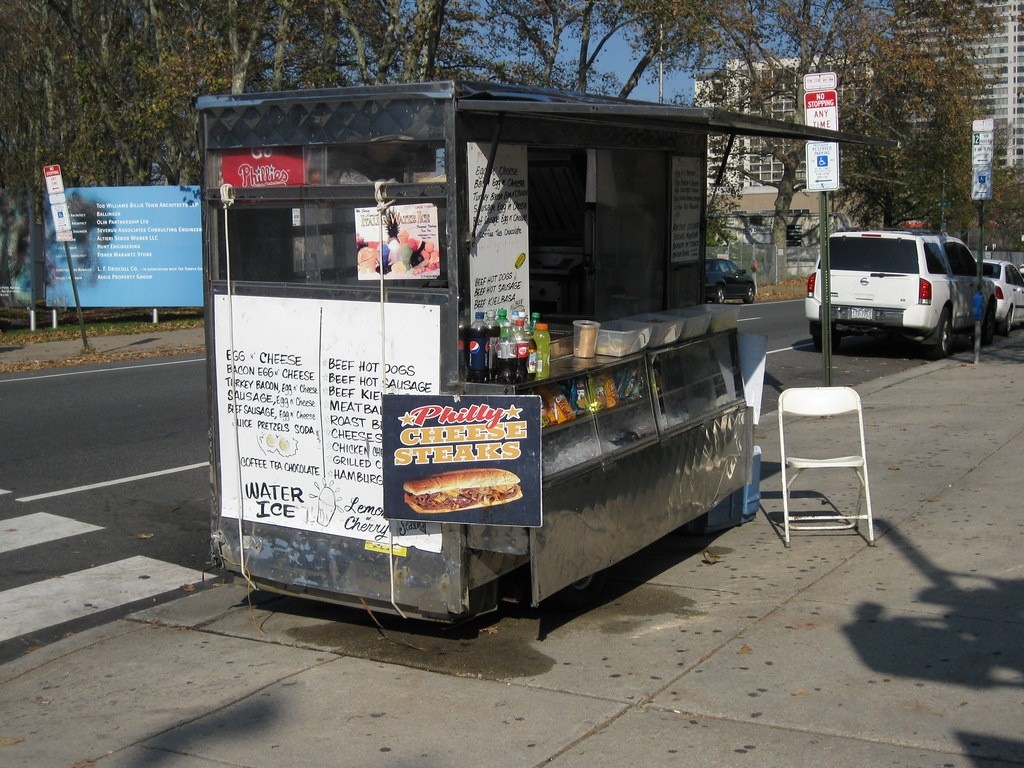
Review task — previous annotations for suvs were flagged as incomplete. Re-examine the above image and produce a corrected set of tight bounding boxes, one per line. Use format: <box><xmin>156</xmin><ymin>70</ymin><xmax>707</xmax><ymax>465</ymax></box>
<box><xmin>804</xmin><ymin>230</ymin><xmax>998</xmax><ymax>360</ymax></box>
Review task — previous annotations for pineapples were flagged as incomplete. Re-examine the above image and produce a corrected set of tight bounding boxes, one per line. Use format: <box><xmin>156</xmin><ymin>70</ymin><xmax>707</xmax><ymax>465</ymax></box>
<box><xmin>383</xmin><ymin>209</ymin><xmax>400</xmax><ymax>253</ymax></box>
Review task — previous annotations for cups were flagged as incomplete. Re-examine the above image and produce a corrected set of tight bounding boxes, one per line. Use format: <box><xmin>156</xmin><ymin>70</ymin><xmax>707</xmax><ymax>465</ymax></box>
<box><xmin>573</xmin><ymin>320</ymin><xmax>601</xmax><ymax>358</ymax></box>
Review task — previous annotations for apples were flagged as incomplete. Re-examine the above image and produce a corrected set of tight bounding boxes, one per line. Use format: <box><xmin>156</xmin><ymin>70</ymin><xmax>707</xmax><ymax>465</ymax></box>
<box><xmin>397</xmin><ymin>230</ymin><xmax>439</xmax><ymax>261</ymax></box>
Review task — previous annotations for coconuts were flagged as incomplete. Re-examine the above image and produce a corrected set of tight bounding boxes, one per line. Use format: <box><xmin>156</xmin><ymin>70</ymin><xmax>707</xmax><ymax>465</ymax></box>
<box><xmin>396</xmin><ymin>243</ymin><xmax>413</xmax><ymax>266</ymax></box>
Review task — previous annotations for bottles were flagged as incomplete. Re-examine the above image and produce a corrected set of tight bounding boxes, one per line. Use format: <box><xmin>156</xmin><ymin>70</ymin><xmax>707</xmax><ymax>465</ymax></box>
<box><xmin>468</xmin><ymin>308</ymin><xmax>551</xmax><ymax>383</ymax></box>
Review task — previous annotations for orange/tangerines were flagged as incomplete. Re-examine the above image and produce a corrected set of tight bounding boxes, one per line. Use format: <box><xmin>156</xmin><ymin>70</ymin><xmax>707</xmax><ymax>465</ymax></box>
<box><xmin>391</xmin><ymin>261</ymin><xmax>406</xmax><ymax>274</ymax></box>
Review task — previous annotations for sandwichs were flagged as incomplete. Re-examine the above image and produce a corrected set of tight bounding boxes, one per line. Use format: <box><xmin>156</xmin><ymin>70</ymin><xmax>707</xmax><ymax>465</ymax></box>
<box><xmin>403</xmin><ymin>468</ymin><xmax>523</xmax><ymax>514</ymax></box>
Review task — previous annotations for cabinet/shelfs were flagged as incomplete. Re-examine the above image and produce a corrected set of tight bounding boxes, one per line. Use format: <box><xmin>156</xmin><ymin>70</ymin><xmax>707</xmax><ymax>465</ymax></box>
<box><xmin>462</xmin><ymin>327</ymin><xmax>753</xmax><ymax>608</ymax></box>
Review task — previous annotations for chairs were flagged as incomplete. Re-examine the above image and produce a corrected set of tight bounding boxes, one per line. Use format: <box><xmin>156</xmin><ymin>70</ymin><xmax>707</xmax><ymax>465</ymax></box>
<box><xmin>983</xmin><ymin>264</ymin><xmax>993</xmax><ymax>277</ymax></box>
<box><xmin>778</xmin><ymin>386</ymin><xmax>874</xmax><ymax>547</ymax></box>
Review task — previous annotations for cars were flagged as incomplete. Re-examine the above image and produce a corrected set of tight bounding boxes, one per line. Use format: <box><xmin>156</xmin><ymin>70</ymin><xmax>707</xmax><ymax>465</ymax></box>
<box><xmin>974</xmin><ymin>258</ymin><xmax>1024</xmax><ymax>338</ymax></box>
<box><xmin>703</xmin><ymin>258</ymin><xmax>756</xmax><ymax>305</ymax></box>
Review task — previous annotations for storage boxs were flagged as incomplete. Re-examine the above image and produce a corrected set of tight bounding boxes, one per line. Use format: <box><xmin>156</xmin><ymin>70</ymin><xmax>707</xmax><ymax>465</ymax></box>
<box><xmin>597</xmin><ymin>303</ymin><xmax>741</xmax><ymax>356</ymax></box>
<box><xmin>688</xmin><ymin>446</ymin><xmax>761</xmax><ymax>535</ymax></box>
